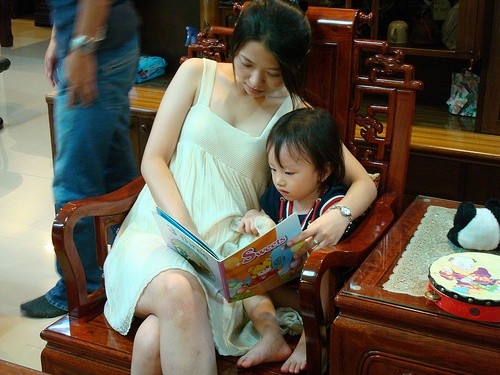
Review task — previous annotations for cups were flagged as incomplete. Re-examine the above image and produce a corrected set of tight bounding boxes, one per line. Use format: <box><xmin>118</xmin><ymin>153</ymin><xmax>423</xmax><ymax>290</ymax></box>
<box><xmin>387</xmin><ymin>20</ymin><xmax>409</xmax><ymax>44</ymax></box>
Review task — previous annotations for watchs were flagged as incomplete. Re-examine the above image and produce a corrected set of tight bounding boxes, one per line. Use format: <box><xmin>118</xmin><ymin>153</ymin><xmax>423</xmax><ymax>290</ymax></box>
<box><xmin>328</xmin><ymin>205</ymin><xmax>353</xmax><ymax>235</ymax></box>
<box><xmin>68</xmin><ymin>34</ymin><xmax>106</xmax><ymax>52</ymax></box>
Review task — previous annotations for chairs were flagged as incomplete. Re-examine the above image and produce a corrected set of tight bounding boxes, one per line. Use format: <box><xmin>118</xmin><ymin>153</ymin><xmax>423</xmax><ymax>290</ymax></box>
<box><xmin>40</xmin><ymin>6</ymin><xmax>422</xmax><ymax>375</ymax></box>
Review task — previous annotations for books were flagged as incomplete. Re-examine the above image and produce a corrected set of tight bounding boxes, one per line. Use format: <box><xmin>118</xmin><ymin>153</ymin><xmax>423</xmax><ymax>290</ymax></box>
<box><xmin>152</xmin><ymin>207</ymin><xmax>310</xmax><ymax>303</ymax></box>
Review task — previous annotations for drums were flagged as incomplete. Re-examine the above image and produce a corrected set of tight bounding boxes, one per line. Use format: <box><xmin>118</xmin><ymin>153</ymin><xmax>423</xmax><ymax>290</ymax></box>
<box><xmin>423</xmin><ymin>251</ymin><xmax>500</xmax><ymax>321</ymax></box>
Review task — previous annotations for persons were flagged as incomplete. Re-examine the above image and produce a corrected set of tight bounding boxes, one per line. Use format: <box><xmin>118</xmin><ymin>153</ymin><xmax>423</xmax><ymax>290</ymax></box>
<box><xmin>236</xmin><ymin>106</ymin><xmax>350</xmax><ymax>373</ymax></box>
<box><xmin>103</xmin><ymin>1</ymin><xmax>378</xmax><ymax>375</ymax></box>
<box><xmin>19</xmin><ymin>0</ymin><xmax>140</xmax><ymax>318</ymax></box>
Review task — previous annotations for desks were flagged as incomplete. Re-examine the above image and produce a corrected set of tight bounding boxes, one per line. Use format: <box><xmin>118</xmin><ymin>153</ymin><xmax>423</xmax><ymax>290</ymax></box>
<box><xmin>329</xmin><ymin>193</ymin><xmax>500</xmax><ymax>375</ymax></box>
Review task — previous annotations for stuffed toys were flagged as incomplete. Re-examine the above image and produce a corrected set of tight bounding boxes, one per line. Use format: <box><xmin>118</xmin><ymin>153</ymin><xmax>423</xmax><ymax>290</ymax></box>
<box><xmin>447</xmin><ymin>202</ymin><xmax>499</xmax><ymax>252</ymax></box>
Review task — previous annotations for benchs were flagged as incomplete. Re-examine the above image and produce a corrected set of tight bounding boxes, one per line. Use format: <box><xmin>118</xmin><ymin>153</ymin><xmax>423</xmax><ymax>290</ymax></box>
<box><xmin>44</xmin><ymin>79</ymin><xmax>500</xmax><ymax>169</ymax></box>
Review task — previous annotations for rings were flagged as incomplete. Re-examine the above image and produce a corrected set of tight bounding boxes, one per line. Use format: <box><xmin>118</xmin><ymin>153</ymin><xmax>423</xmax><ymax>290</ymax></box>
<box><xmin>312</xmin><ymin>238</ymin><xmax>320</xmax><ymax>245</ymax></box>
